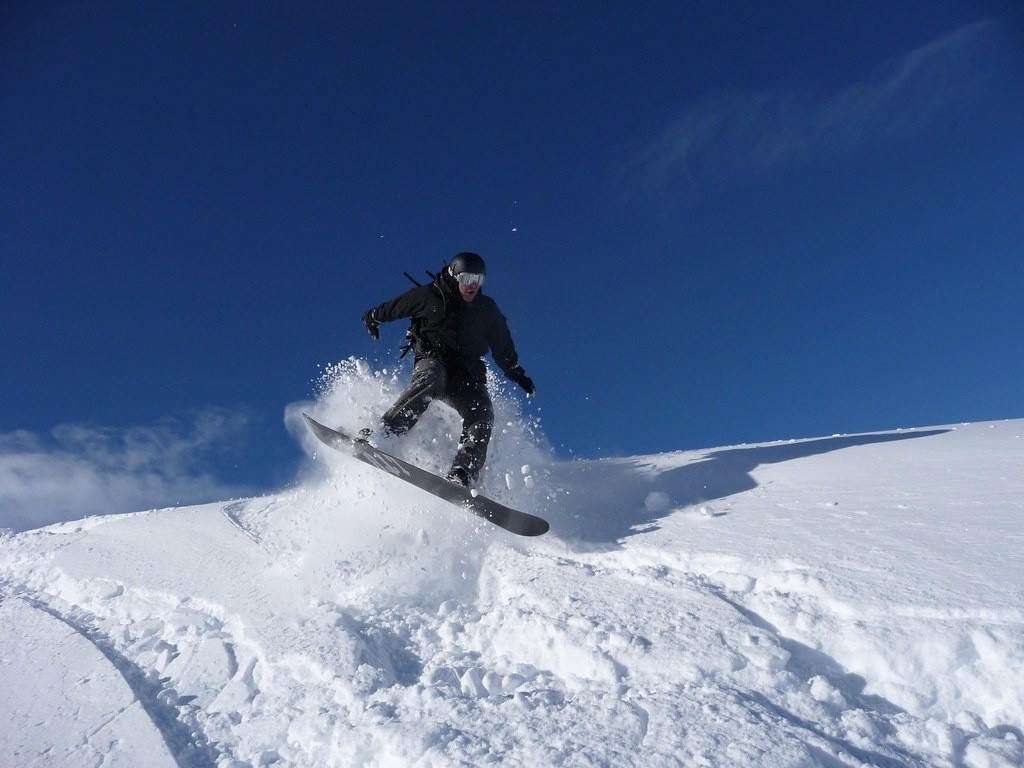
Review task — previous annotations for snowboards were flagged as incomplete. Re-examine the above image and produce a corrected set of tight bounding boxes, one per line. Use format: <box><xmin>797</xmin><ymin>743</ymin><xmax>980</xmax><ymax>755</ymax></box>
<box><xmin>302</xmin><ymin>412</ymin><xmax>550</xmax><ymax>537</ymax></box>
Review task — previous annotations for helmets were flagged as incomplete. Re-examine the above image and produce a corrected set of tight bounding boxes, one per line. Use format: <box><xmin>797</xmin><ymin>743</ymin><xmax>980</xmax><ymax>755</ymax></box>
<box><xmin>449</xmin><ymin>252</ymin><xmax>486</xmax><ymax>287</ymax></box>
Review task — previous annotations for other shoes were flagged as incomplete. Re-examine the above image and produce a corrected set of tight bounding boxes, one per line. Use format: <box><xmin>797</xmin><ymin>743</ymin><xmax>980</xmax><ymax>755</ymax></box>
<box><xmin>445</xmin><ymin>467</ymin><xmax>469</xmax><ymax>486</ymax></box>
<box><xmin>358</xmin><ymin>426</ymin><xmax>389</xmax><ymax>446</ymax></box>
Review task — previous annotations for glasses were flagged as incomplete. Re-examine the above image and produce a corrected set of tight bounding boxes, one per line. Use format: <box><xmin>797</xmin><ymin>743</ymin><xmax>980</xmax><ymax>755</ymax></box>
<box><xmin>455</xmin><ymin>272</ymin><xmax>485</xmax><ymax>287</ymax></box>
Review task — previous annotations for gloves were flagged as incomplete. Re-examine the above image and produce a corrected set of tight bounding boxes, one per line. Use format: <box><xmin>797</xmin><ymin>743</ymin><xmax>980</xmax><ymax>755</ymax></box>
<box><xmin>360</xmin><ymin>308</ymin><xmax>381</xmax><ymax>340</ymax></box>
<box><xmin>504</xmin><ymin>366</ymin><xmax>537</xmax><ymax>394</ymax></box>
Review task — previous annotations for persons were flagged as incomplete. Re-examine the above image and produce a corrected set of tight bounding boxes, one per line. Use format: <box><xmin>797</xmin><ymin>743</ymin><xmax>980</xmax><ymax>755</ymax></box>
<box><xmin>362</xmin><ymin>253</ymin><xmax>535</xmax><ymax>486</ymax></box>
